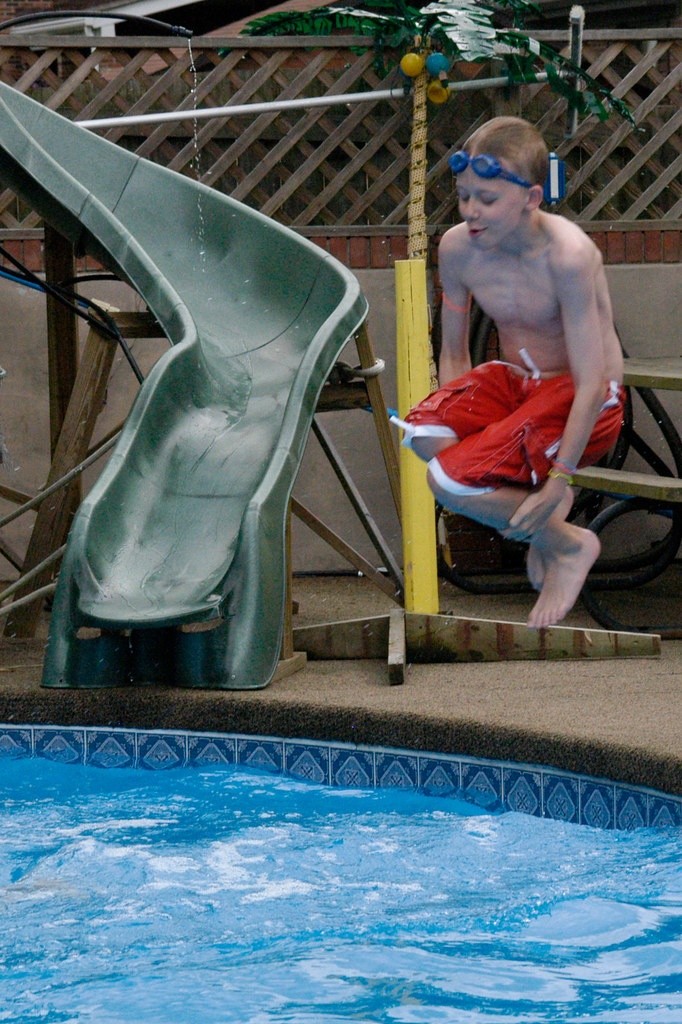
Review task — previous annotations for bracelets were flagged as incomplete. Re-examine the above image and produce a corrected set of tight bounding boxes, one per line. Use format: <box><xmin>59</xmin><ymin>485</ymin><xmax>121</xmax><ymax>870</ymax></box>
<box><xmin>441</xmin><ymin>290</ymin><xmax>472</xmax><ymax>313</ymax></box>
<box><xmin>547</xmin><ymin>458</ymin><xmax>578</xmax><ymax>486</ymax></box>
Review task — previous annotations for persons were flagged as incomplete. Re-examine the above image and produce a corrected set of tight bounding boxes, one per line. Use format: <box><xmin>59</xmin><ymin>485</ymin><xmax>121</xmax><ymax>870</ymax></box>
<box><xmin>401</xmin><ymin>114</ymin><xmax>627</xmax><ymax>630</ymax></box>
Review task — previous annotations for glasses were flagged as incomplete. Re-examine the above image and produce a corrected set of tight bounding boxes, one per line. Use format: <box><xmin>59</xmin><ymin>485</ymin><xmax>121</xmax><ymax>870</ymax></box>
<box><xmin>448</xmin><ymin>151</ymin><xmax>532</xmax><ymax>189</ymax></box>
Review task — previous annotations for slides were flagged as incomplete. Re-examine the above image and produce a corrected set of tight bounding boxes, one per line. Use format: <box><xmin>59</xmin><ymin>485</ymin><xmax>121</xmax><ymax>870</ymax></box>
<box><xmin>0</xmin><ymin>80</ymin><xmax>374</xmax><ymax>692</ymax></box>
